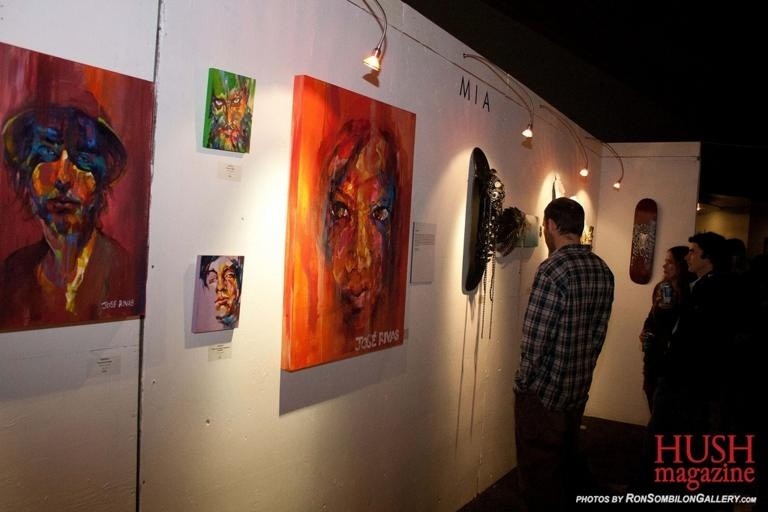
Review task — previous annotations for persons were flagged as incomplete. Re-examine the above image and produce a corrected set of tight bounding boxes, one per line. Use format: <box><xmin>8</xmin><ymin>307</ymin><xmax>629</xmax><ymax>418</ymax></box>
<box><xmin>198</xmin><ymin>255</ymin><xmax>244</xmax><ymax>329</ymax></box>
<box><xmin>1</xmin><ymin>74</ymin><xmax>143</xmax><ymax>332</ymax></box>
<box><xmin>512</xmin><ymin>198</ymin><xmax>614</xmax><ymax>510</ymax></box>
<box><xmin>318</xmin><ymin>114</ymin><xmax>402</xmax><ymax>350</ymax></box>
<box><xmin>208</xmin><ymin>70</ymin><xmax>254</xmax><ymax>154</ymax></box>
<box><xmin>640</xmin><ymin>232</ymin><xmax>767</xmax><ymax>512</ymax></box>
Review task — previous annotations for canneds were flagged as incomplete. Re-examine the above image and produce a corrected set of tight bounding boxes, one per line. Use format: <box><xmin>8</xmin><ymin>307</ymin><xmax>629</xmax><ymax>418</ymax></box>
<box><xmin>661</xmin><ymin>282</ymin><xmax>673</xmax><ymax>304</ymax></box>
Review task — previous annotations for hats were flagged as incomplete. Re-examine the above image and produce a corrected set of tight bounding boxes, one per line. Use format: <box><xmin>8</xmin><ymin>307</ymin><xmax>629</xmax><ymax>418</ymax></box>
<box><xmin>0</xmin><ymin>84</ymin><xmax>129</xmax><ymax>187</ymax></box>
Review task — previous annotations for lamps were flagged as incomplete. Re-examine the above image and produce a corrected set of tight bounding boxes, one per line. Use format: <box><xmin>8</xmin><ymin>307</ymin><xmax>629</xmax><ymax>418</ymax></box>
<box><xmin>465</xmin><ymin>54</ymin><xmax>535</xmax><ymax>137</ymax></box>
<box><xmin>362</xmin><ymin>0</ymin><xmax>387</xmax><ymax>71</ymax></box>
<box><xmin>541</xmin><ymin>103</ymin><xmax>589</xmax><ymax>177</ymax></box>
<box><xmin>585</xmin><ymin>134</ymin><xmax>625</xmax><ymax>189</ymax></box>
